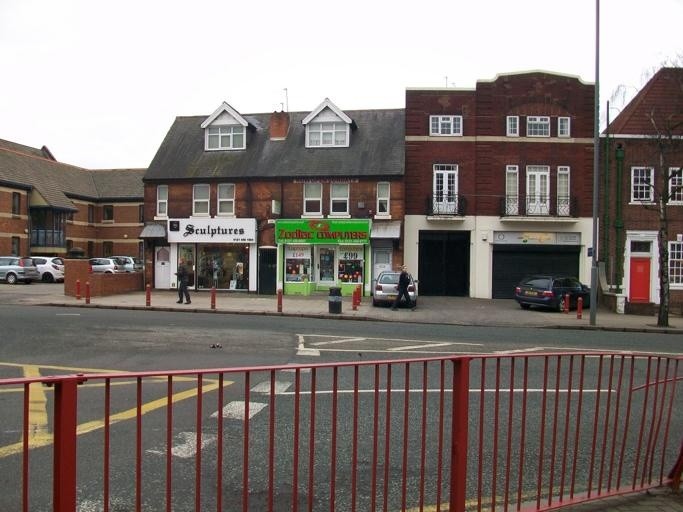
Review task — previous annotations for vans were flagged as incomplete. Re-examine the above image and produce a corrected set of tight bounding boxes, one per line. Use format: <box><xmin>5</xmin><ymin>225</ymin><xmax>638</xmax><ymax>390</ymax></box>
<box><xmin>89</xmin><ymin>258</ymin><xmax>124</xmax><ymax>275</ymax></box>
<box><xmin>30</xmin><ymin>257</ymin><xmax>65</xmax><ymax>283</ymax></box>
<box><xmin>108</xmin><ymin>255</ymin><xmax>143</xmax><ymax>275</ymax></box>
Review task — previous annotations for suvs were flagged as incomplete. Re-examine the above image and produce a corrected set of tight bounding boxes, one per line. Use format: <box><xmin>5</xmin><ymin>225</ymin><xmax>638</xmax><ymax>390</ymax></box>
<box><xmin>0</xmin><ymin>256</ymin><xmax>40</xmax><ymax>285</ymax></box>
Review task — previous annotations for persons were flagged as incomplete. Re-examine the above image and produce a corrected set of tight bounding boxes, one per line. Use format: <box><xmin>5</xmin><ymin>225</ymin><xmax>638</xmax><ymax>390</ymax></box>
<box><xmin>391</xmin><ymin>265</ymin><xmax>416</xmax><ymax>311</ymax></box>
<box><xmin>174</xmin><ymin>257</ymin><xmax>191</xmax><ymax>304</ymax></box>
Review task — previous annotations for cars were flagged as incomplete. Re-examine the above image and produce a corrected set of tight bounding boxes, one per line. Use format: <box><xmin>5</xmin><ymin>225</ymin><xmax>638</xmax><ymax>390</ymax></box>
<box><xmin>370</xmin><ymin>271</ymin><xmax>419</xmax><ymax>308</ymax></box>
<box><xmin>514</xmin><ymin>273</ymin><xmax>591</xmax><ymax>311</ymax></box>
<box><xmin>198</xmin><ymin>255</ymin><xmax>222</xmax><ymax>287</ymax></box>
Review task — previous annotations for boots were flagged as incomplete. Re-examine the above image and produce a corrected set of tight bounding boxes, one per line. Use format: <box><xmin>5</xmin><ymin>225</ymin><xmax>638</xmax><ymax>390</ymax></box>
<box><xmin>176</xmin><ymin>293</ymin><xmax>184</xmax><ymax>304</ymax></box>
<box><xmin>182</xmin><ymin>293</ymin><xmax>192</xmax><ymax>304</ymax></box>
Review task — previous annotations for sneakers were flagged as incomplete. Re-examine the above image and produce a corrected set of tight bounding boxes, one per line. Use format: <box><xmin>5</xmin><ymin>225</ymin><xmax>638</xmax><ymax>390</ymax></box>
<box><xmin>391</xmin><ymin>307</ymin><xmax>400</xmax><ymax>311</ymax></box>
<box><xmin>410</xmin><ymin>306</ymin><xmax>417</xmax><ymax>311</ymax></box>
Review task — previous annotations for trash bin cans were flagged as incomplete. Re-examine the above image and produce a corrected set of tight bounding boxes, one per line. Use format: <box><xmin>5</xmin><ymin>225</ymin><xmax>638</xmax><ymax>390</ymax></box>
<box><xmin>328</xmin><ymin>287</ymin><xmax>342</xmax><ymax>313</ymax></box>
